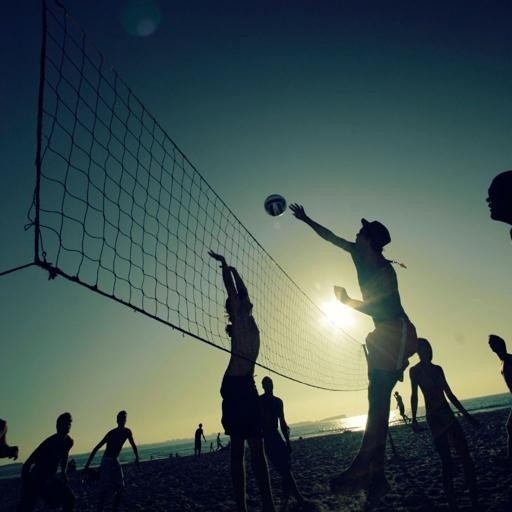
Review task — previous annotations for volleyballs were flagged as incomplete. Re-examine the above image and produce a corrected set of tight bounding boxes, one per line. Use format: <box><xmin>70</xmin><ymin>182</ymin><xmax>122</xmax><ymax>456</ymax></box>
<box><xmin>263</xmin><ymin>195</ymin><xmax>286</xmax><ymax>215</ymax></box>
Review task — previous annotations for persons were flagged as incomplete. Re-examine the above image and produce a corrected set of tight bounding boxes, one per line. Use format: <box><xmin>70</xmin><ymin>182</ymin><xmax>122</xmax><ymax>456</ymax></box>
<box><xmin>210</xmin><ymin>432</ymin><xmax>223</xmax><ymax>452</ymax></box>
<box><xmin>410</xmin><ymin>337</ymin><xmax>480</xmax><ymax>512</ymax></box>
<box><xmin>150</xmin><ymin>453</ymin><xmax>182</xmax><ymax>466</ymax></box>
<box><xmin>1</xmin><ymin>419</ymin><xmax>19</xmax><ymax>461</ymax></box>
<box><xmin>486</xmin><ymin>167</ymin><xmax>511</xmax><ymax>241</ymax></box>
<box><xmin>67</xmin><ymin>459</ymin><xmax>76</xmax><ymax>487</ymax></box>
<box><xmin>457</xmin><ymin>413</ymin><xmax>465</xmax><ymax>425</ymax></box>
<box><xmin>195</xmin><ymin>423</ymin><xmax>206</xmax><ymax>457</ymax></box>
<box><xmin>80</xmin><ymin>410</ymin><xmax>141</xmax><ymax>512</ymax></box>
<box><xmin>207</xmin><ymin>248</ymin><xmax>275</xmax><ymax>511</ymax></box>
<box><xmin>20</xmin><ymin>412</ymin><xmax>74</xmax><ymax>512</ymax></box>
<box><xmin>394</xmin><ymin>391</ymin><xmax>411</xmax><ymax>424</ymax></box>
<box><xmin>488</xmin><ymin>334</ymin><xmax>512</xmax><ymax>466</ymax></box>
<box><xmin>288</xmin><ymin>203</ymin><xmax>416</xmax><ymax>510</ymax></box>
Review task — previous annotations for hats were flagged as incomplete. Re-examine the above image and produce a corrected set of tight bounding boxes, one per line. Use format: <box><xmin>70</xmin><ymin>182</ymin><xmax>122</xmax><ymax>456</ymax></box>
<box><xmin>361</xmin><ymin>218</ymin><xmax>391</xmax><ymax>247</ymax></box>
